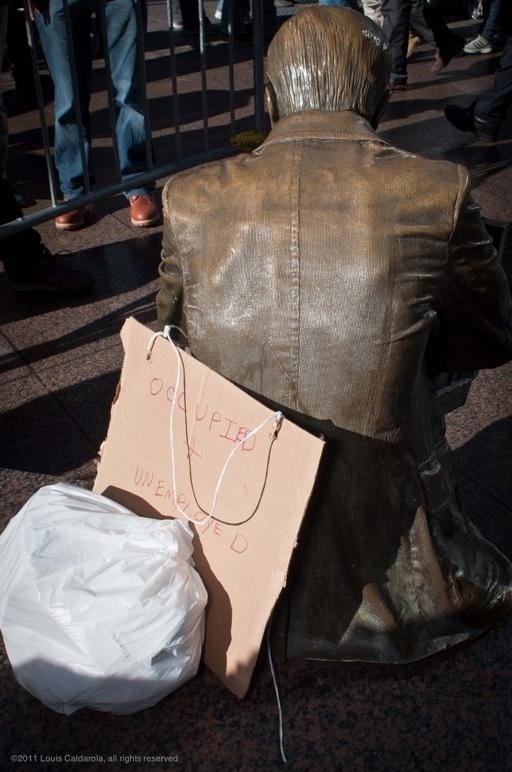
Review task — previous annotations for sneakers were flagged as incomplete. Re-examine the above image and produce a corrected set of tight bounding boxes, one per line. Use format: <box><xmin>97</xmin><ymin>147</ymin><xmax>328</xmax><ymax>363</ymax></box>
<box><xmin>388</xmin><ymin>2</ymin><xmax>494</xmax><ymax>91</ymax></box>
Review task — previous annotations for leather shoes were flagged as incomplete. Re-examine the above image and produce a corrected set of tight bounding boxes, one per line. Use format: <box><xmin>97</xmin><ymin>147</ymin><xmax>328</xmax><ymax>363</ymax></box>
<box><xmin>56</xmin><ymin>202</ymin><xmax>94</xmax><ymax>231</ymax></box>
<box><xmin>445</xmin><ymin>104</ymin><xmax>499</xmax><ymax>141</ymax></box>
<box><xmin>128</xmin><ymin>195</ymin><xmax>162</xmax><ymax>227</ymax></box>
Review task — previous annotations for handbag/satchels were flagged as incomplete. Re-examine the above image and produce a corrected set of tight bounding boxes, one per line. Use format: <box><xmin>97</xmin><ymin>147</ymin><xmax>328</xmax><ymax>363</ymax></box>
<box><xmin>95</xmin><ymin>313</ymin><xmax>328</xmax><ymax>700</ymax></box>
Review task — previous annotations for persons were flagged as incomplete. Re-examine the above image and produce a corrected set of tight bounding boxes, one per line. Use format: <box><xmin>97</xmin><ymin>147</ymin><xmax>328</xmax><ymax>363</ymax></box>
<box><xmin>35</xmin><ymin>1</ymin><xmax>161</xmax><ymax>232</ymax></box>
<box><xmin>156</xmin><ymin>6</ymin><xmax>511</xmax><ymax>664</ymax></box>
<box><xmin>1</xmin><ymin>1</ymin><xmax>510</xmax><ymax>141</ymax></box>
